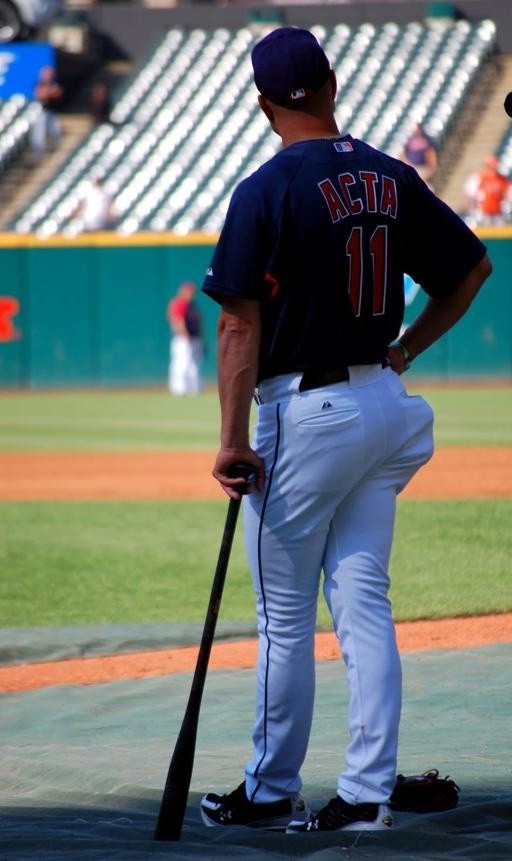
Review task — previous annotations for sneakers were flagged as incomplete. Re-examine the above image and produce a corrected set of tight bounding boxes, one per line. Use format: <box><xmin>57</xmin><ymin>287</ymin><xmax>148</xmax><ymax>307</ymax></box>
<box><xmin>282</xmin><ymin>794</ymin><xmax>396</xmax><ymax>837</ymax></box>
<box><xmin>199</xmin><ymin>780</ymin><xmax>313</xmax><ymax>830</ymax></box>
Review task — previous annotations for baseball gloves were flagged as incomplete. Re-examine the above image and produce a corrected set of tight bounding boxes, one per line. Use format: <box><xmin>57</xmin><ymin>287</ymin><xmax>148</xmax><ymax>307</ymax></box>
<box><xmin>389</xmin><ymin>769</ymin><xmax>460</xmax><ymax>813</ymax></box>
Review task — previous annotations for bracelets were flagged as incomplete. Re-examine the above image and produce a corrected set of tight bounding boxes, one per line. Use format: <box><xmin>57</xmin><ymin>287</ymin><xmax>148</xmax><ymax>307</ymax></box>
<box><xmin>388</xmin><ymin>339</ymin><xmax>413</xmax><ymax>371</ymax></box>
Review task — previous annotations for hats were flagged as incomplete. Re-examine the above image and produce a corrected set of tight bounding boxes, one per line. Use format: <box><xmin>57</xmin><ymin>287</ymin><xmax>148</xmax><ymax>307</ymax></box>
<box><xmin>248</xmin><ymin>26</ymin><xmax>329</xmax><ymax>110</ymax></box>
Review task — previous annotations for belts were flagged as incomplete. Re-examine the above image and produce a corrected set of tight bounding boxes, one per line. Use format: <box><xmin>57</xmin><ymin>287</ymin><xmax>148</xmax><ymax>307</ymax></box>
<box><xmin>298</xmin><ymin>364</ymin><xmax>350</xmax><ymax>393</ymax></box>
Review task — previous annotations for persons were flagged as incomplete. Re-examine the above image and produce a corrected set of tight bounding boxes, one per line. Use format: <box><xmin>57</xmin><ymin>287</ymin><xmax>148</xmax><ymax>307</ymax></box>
<box><xmin>465</xmin><ymin>155</ymin><xmax>512</xmax><ymax>229</ymax></box>
<box><xmin>403</xmin><ymin>123</ymin><xmax>440</xmax><ymax>191</ymax></box>
<box><xmin>162</xmin><ymin>281</ymin><xmax>205</xmax><ymax>401</ymax></box>
<box><xmin>198</xmin><ymin>25</ymin><xmax>495</xmax><ymax>838</ymax></box>
<box><xmin>73</xmin><ymin>199</ymin><xmax>121</xmax><ymax>236</ymax></box>
<box><xmin>1</xmin><ymin>0</ymin><xmax>134</xmax><ymax>142</ymax></box>
<box><xmin>65</xmin><ymin>172</ymin><xmax>108</xmax><ymax>235</ymax></box>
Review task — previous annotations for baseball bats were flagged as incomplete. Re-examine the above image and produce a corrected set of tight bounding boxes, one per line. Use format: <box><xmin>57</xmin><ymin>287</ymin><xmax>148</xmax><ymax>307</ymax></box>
<box><xmin>154</xmin><ymin>461</ymin><xmax>253</xmax><ymax>840</ymax></box>
